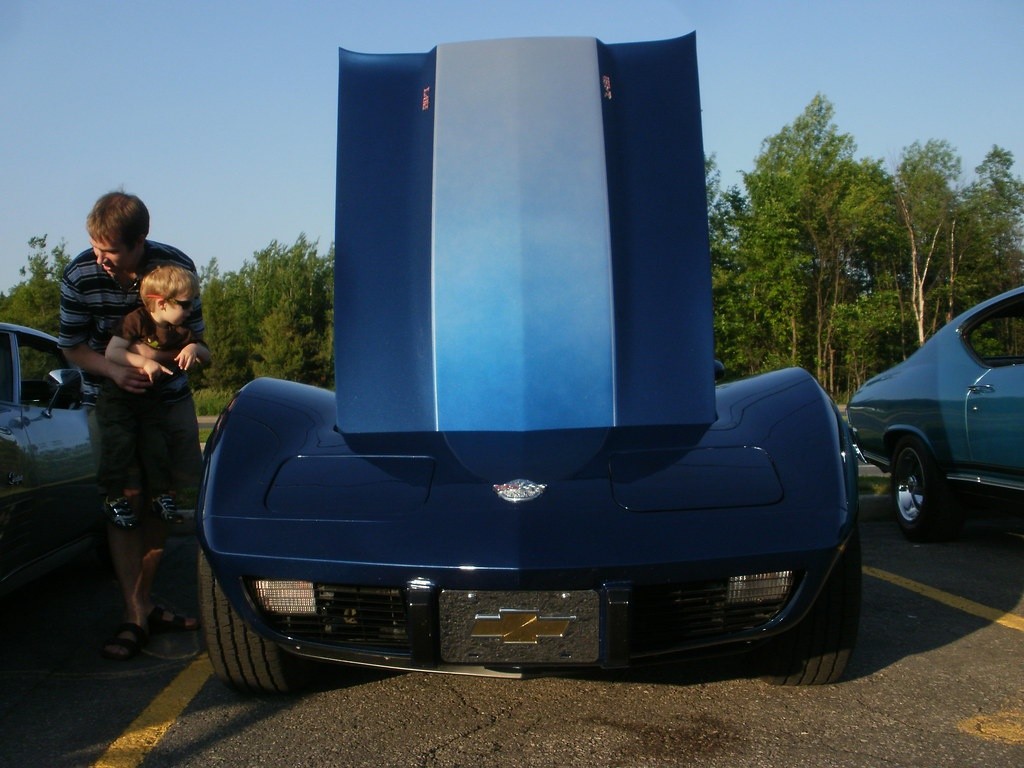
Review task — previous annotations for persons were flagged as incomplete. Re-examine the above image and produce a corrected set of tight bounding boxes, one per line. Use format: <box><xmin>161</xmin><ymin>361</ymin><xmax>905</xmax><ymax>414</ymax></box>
<box><xmin>94</xmin><ymin>266</ymin><xmax>212</xmax><ymax>530</ymax></box>
<box><xmin>58</xmin><ymin>192</ymin><xmax>204</xmax><ymax>659</ymax></box>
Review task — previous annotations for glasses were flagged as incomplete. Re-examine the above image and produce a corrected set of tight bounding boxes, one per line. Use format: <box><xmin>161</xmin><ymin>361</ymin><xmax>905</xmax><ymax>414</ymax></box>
<box><xmin>145</xmin><ymin>293</ymin><xmax>196</xmax><ymax>310</ymax></box>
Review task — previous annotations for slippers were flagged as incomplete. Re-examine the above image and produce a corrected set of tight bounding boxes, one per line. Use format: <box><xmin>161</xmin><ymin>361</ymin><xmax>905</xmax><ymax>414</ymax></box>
<box><xmin>144</xmin><ymin>607</ymin><xmax>200</xmax><ymax>634</ymax></box>
<box><xmin>101</xmin><ymin>621</ymin><xmax>152</xmax><ymax>661</ymax></box>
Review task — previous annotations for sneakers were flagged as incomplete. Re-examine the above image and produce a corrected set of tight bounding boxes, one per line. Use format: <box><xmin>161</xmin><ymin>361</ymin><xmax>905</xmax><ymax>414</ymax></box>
<box><xmin>151</xmin><ymin>495</ymin><xmax>184</xmax><ymax>524</ymax></box>
<box><xmin>101</xmin><ymin>494</ymin><xmax>138</xmax><ymax>529</ymax></box>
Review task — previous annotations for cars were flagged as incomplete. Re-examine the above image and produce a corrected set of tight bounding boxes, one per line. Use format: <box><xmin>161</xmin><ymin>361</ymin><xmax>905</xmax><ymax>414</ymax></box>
<box><xmin>0</xmin><ymin>320</ymin><xmax>102</xmax><ymax>591</ymax></box>
<box><xmin>846</xmin><ymin>287</ymin><xmax>1024</xmax><ymax>542</ymax></box>
<box><xmin>192</xmin><ymin>30</ymin><xmax>862</xmax><ymax>697</ymax></box>
